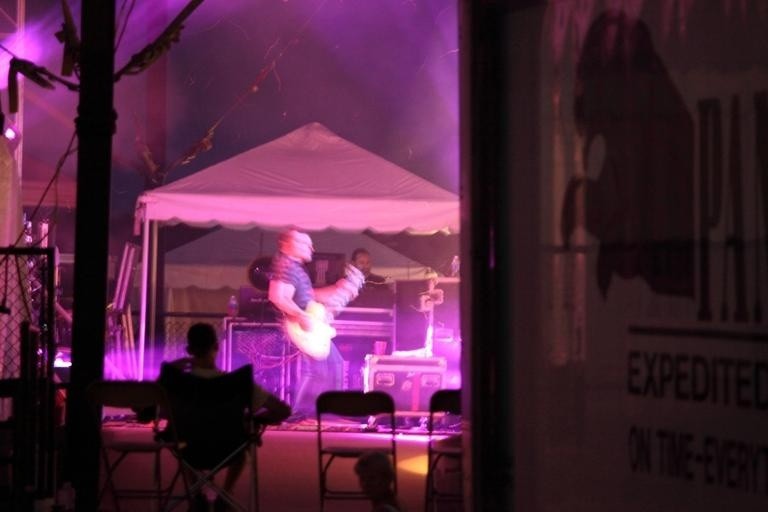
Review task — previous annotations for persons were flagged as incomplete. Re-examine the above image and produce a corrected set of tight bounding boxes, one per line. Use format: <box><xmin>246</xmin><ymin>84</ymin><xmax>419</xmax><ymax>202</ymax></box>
<box><xmin>168</xmin><ymin>323</ymin><xmax>292</xmax><ymax>511</ymax></box>
<box><xmin>350</xmin><ymin>250</ymin><xmax>395</xmax><ymax>313</ymax></box>
<box><xmin>268</xmin><ymin>232</ymin><xmax>345</xmax><ymax>424</ymax></box>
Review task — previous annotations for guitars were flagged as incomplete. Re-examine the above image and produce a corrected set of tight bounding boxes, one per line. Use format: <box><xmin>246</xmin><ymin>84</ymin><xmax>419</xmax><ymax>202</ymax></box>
<box><xmin>284</xmin><ymin>264</ymin><xmax>365</xmax><ymax>360</ymax></box>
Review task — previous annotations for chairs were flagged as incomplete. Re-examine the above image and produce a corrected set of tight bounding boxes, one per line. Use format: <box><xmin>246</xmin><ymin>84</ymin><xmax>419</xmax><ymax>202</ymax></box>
<box><xmin>316</xmin><ymin>390</ymin><xmax>397</xmax><ymax>511</ymax></box>
<box><xmin>98</xmin><ymin>364</ymin><xmax>282</xmax><ymax>512</ymax></box>
<box><xmin>425</xmin><ymin>390</ymin><xmax>464</xmax><ymax>512</ymax></box>
